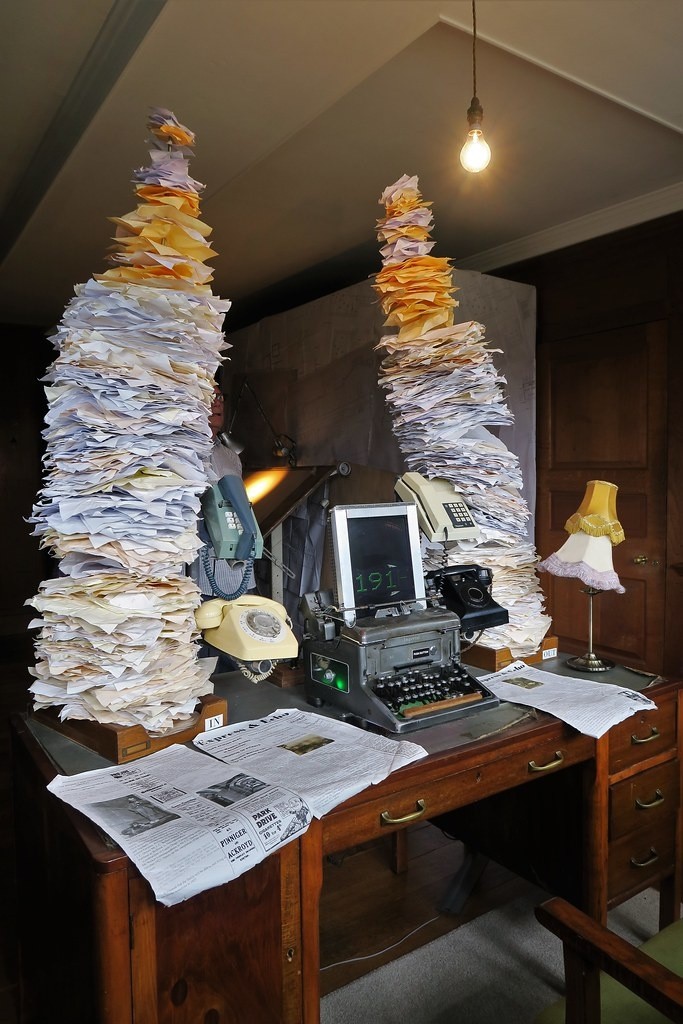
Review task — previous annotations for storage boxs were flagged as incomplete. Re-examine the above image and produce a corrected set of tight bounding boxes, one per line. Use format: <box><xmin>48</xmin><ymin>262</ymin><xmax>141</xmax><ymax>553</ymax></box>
<box><xmin>27</xmin><ymin>697</ymin><xmax>228</xmax><ymax>764</ymax></box>
<box><xmin>459</xmin><ymin>636</ymin><xmax>558</xmax><ymax>671</ymax></box>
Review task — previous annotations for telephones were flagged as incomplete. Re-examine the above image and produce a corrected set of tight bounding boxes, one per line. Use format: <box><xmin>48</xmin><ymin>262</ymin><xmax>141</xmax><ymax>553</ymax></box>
<box><xmin>199</xmin><ymin>474</ymin><xmax>265</xmax><ymax>560</ymax></box>
<box><xmin>394</xmin><ymin>471</ymin><xmax>480</xmax><ymax>542</ymax></box>
<box><xmin>193</xmin><ymin>593</ymin><xmax>299</xmax><ymax>664</ymax></box>
<box><xmin>424</xmin><ymin>564</ymin><xmax>510</xmax><ymax>634</ymax></box>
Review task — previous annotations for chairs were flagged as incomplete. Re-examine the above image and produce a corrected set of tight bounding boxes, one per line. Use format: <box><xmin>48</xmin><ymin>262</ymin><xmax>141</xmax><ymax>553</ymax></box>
<box><xmin>534</xmin><ymin>896</ymin><xmax>683</xmax><ymax>1024</ymax></box>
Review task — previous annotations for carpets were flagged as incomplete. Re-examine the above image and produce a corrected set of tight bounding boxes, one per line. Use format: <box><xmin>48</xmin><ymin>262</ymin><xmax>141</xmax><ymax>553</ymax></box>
<box><xmin>320</xmin><ymin>887</ymin><xmax>683</xmax><ymax>1024</ymax></box>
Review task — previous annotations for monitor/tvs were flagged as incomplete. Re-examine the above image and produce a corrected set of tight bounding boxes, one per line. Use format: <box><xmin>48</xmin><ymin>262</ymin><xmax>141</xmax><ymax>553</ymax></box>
<box><xmin>321</xmin><ymin>504</ymin><xmax>427</xmax><ymax>625</ymax></box>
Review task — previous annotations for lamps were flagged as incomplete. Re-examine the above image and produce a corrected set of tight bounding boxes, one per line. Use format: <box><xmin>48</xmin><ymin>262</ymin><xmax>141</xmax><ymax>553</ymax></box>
<box><xmin>542</xmin><ymin>481</ymin><xmax>627</xmax><ymax>671</ymax></box>
<box><xmin>217</xmin><ymin>381</ymin><xmax>299</xmax><ymax>466</ymax></box>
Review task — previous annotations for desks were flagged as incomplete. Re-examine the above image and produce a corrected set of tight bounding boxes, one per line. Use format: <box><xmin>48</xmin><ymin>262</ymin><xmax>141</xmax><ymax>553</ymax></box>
<box><xmin>24</xmin><ymin>653</ymin><xmax>683</xmax><ymax>1024</ymax></box>
